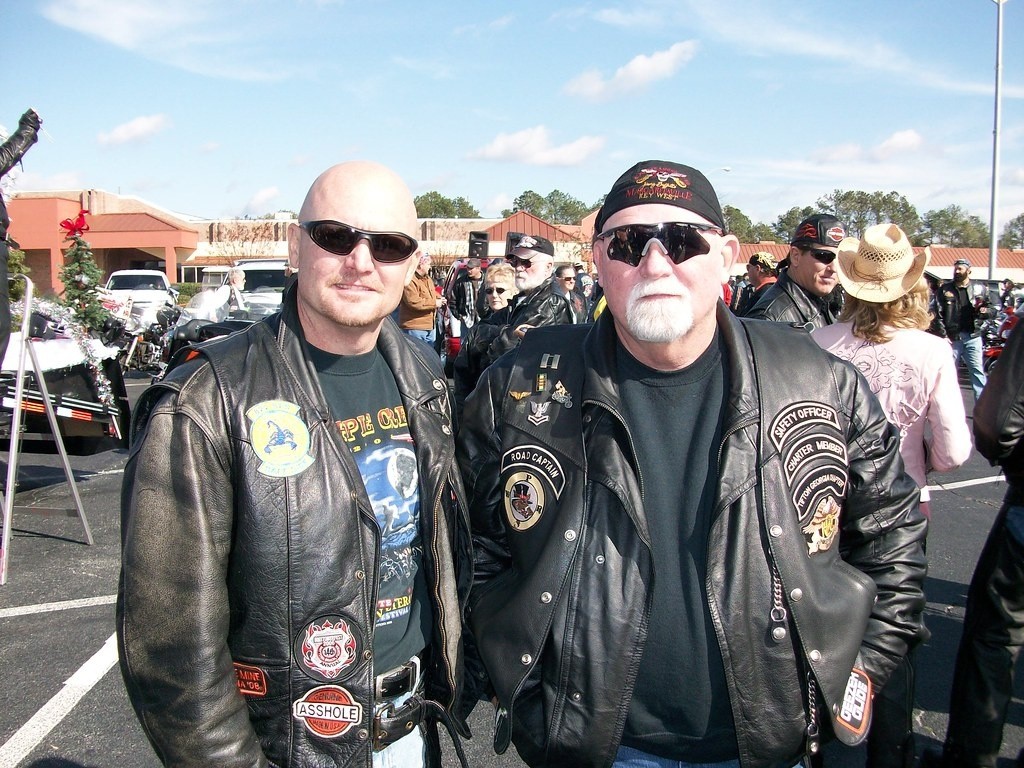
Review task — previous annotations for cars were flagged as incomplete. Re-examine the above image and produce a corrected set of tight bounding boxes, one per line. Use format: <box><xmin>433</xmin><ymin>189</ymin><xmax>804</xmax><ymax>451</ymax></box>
<box><xmin>210</xmin><ymin>261</ymin><xmax>288</xmax><ymax>320</ymax></box>
<box><xmin>104</xmin><ymin>270</ymin><xmax>175</xmax><ymax>310</ymax></box>
<box><xmin>971</xmin><ymin>280</ymin><xmax>1024</xmax><ymax>309</ymax></box>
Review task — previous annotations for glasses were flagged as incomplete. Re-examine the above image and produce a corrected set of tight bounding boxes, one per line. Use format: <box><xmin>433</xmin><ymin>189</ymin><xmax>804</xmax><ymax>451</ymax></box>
<box><xmin>482</xmin><ymin>287</ymin><xmax>512</xmax><ymax>294</ymax></box>
<box><xmin>1003</xmin><ymin>282</ymin><xmax>1007</xmax><ymax>284</ymax></box>
<box><xmin>595</xmin><ymin>221</ymin><xmax>723</xmax><ymax>267</ymax></box>
<box><xmin>299</xmin><ymin>219</ymin><xmax>419</xmax><ymax>264</ymax></box>
<box><xmin>746</xmin><ymin>264</ymin><xmax>750</xmax><ymax>271</ymax></box>
<box><xmin>509</xmin><ymin>259</ymin><xmax>532</xmax><ymax>269</ymax></box>
<box><xmin>799</xmin><ymin>246</ymin><xmax>836</xmax><ymax>265</ymax></box>
<box><xmin>954</xmin><ymin>260</ymin><xmax>970</xmax><ymax>266</ymax></box>
<box><xmin>561</xmin><ymin>276</ymin><xmax>576</xmax><ymax>281</ymax></box>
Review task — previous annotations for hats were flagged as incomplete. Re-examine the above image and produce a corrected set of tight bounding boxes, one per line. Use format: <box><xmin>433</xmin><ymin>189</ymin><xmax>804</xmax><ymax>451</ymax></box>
<box><xmin>954</xmin><ymin>258</ymin><xmax>971</xmax><ymax>268</ymax></box>
<box><xmin>505</xmin><ymin>235</ymin><xmax>554</xmax><ymax>259</ymax></box>
<box><xmin>464</xmin><ymin>257</ymin><xmax>480</xmax><ymax>269</ymax></box>
<box><xmin>1003</xmin><ymin>277</ymin><xmax>1015</xmax><ymax>284</ymax></box>
<box><xmin>419</xmin><ymin>252</ymin><xmax>431</xmax><ymax>265</ymax></box>
<box><xmin>791</xmin><ymin>215</ymin><xmax>850</xmax><ymax>247</ymax></box>
<box><xmin>835</xmin><ymin>222</ymin><xmax>931</xmax><ymax>302</ymax></box>
<box><xmin>595</xmin><ymin>160</ymin><xmax>728</xmax><ymax>233</ymax></box>
<box><xmin>749</xmin><ymin>251</ymin><xmax>778</xmax><ymax>273</ymax></box>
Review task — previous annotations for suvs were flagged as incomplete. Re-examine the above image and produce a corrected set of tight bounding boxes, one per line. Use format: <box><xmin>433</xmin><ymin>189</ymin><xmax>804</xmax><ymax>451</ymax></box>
<box><xmin>437</xmin><ymin>258</ymin><xmax>492</xmax><ymax>379</ymax></box>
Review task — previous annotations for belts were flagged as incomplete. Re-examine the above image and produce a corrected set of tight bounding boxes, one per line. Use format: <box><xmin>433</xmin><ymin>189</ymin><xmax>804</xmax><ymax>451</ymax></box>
<box><xmin>372</xmin><ymin>647</ymin><xmax>431</xmax><ymax>700</ymax></box>
<box><xmin>373</xmin><ymin>691</ymin><xmax>428</xmax><ymax>753</ymax></box>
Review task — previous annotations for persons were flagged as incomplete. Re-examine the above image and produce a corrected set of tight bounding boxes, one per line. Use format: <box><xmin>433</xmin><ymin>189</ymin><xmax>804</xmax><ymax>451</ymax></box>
<box><xmin>282</xmin><ymin>259</ymin><xmax>298</xmax><ymax>308</ymax></box>
<box><xmin>719</xmin><ymin>252</ymin><xmax>782</xmax><ymax>317</ymax></box>
<box><xmin>398</xmin><ymin>235</ymin><xmax>596</xmax><ymax>414</ymax></box>
<box><xmin>940</xmin><ymin>300</ymin><xmax>1024</xmax><ymax>768</ymax></box>
<box><xmin>807</xmin><ymin>223</ymin><xmax>972</xmax><ymax>768</ymax></box>
<box><xmin>116</xmin><ymin>160</ymin><xmax>475</xmax><ymax>768</ymax></box>
<box><xmin>0</xmin><ymin>106</ymin><xmax>42</xmax><ymax>426</ymax></box>
<box><xmin>456</xmin><ymin>160</ymin><xmax>931</xmax><ymax>768</ymax></box>
<box><xmin>933</xmin><ymin>257</ymin><xmax>1022</xmax><ymax>404</ymax></box>
<box><xmin>226</xmin><ymin>269</ymin><xmax>247</xmax><ymax>310</ymax></box>
<box><xmin>745</xmin><ymin>213</ymin><xmax>846</xmax><ymax>328</ymax></box>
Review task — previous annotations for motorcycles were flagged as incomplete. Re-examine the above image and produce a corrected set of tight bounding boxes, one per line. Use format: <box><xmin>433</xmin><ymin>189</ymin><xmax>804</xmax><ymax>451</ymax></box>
<box><xmin>102</xmin><ymin>283</ymin><xmax>248</xmax><ymax>386</ymax></box>
<box><xmin>970</xmin><ymin>281</ymin><xmax>1024</xmax><ymax>378</ymax></box>
